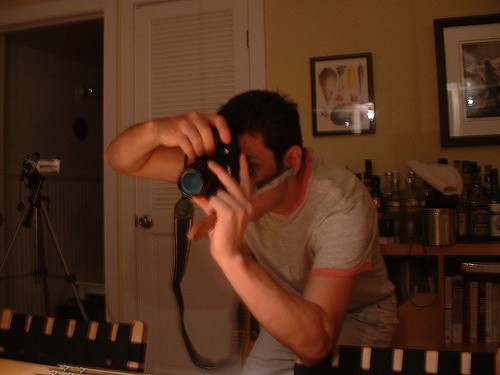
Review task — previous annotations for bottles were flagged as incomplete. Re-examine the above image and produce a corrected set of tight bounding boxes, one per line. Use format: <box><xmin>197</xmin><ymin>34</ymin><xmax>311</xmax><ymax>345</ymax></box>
<box><xmin>353</xmin><ymin>156</ymin><xmax>500</xmax><ymax>244</ymax></box>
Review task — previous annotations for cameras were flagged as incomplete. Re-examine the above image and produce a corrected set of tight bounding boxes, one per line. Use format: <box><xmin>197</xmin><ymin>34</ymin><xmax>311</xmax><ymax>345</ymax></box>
<box><xmin>25</xmin><ymin>158</ymin><xmax>61</xmax><ymax>177</ymax></box>
<box><xmin>179</xmin><ymin>136</ymin><xmax>239</xmax><ymax>197</ymax></box>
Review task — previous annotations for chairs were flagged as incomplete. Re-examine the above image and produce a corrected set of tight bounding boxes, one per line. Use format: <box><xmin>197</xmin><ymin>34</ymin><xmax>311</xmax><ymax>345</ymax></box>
<box><xmin>0</xmin><ymin>308</ymin><xmax>148</xmax><ymax>372</ymax></box>
<box><xmin>294</xmin><ymin>348</ymin><xmax>500</xmax><ymax>375</ymax></box>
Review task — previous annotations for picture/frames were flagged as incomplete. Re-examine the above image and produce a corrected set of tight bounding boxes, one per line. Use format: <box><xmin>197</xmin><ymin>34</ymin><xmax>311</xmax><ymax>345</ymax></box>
<box><xmin>310</xmin><ymin>53</ymin><xmax>376</xmax><ymax>136</ymax></box>
<box><xmin>433</xmin><ymin>13</ymin><xmax>500</xmax><ymax>153</ymax></box>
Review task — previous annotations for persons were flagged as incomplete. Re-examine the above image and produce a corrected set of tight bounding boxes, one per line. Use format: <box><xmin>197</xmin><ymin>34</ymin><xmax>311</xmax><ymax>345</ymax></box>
<box><xmin>103</xmin><ymin>90</ymin><xmax>399</xmax><ymax>375</ymax></box>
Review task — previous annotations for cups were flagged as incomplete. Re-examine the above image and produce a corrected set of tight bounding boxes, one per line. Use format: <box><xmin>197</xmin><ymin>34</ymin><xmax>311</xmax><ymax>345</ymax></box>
<box><xmin>420</xmin><ymin>206</ymin><xmax>457</xmax><ymax>245</ymax></box>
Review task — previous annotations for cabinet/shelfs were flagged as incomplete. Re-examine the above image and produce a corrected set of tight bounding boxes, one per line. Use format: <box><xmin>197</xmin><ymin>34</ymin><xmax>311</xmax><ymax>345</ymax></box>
<box><xmin>241</xmin><ymin>243</ymin><xmax>500</xmax><ymax>367</ymax></box>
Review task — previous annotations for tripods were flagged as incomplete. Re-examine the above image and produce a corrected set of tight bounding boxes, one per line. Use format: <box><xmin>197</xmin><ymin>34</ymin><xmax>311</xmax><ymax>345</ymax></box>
<box><xmin>0</xmin><ymin>180</ymin><xmax>92</xmax><ymax>322</ymax></box>
